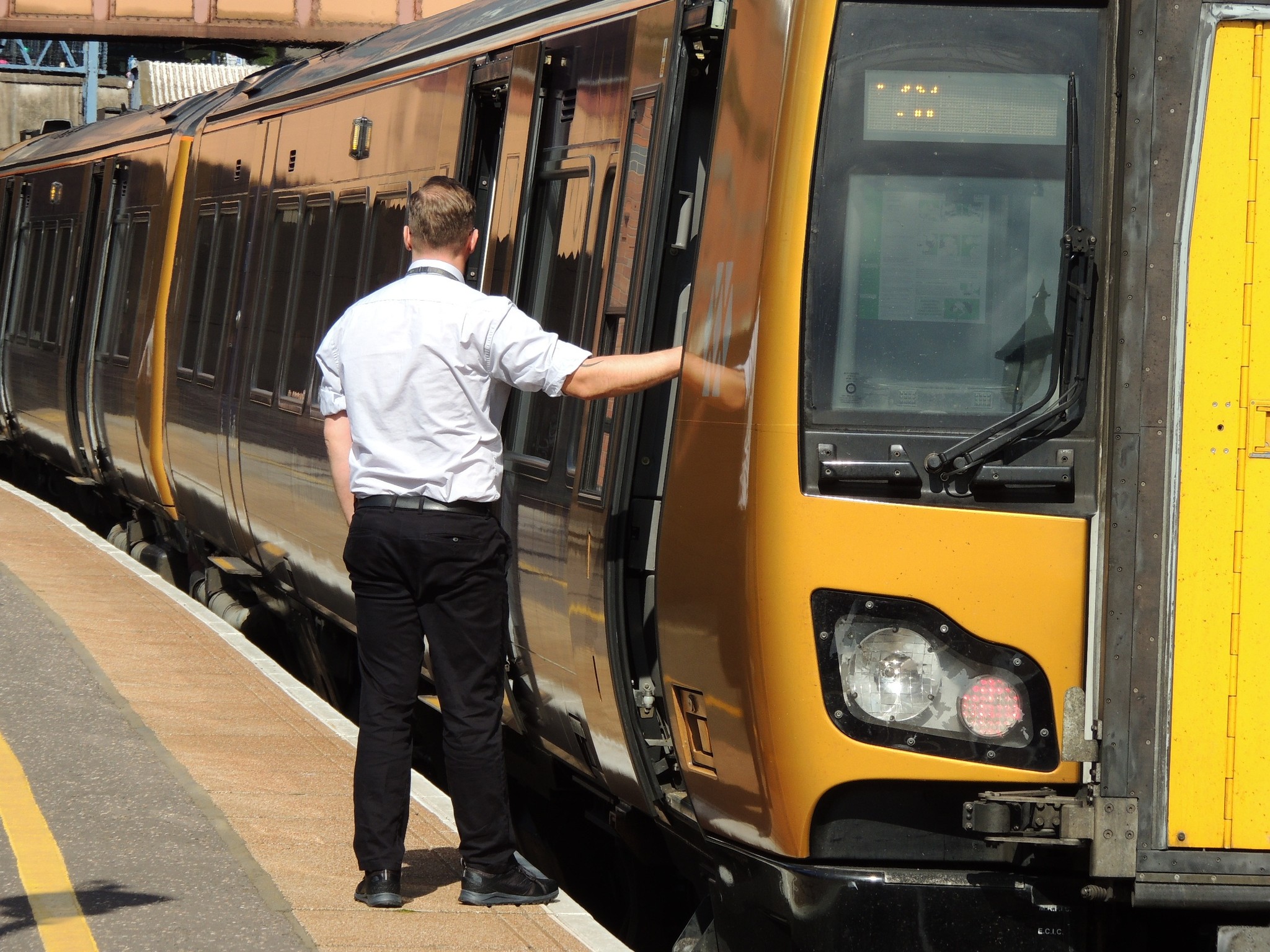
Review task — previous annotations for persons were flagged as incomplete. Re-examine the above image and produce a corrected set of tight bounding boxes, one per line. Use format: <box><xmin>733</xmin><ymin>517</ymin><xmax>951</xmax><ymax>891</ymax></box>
<box><xmin>313</xmin><ymin>177</ymin><xmax>684</xmax><ymax>906</ymax></box>
<box><xmin>684</xmin><ymin>296</ymin><xmax>764</xmax><ymax>515</ymax></box>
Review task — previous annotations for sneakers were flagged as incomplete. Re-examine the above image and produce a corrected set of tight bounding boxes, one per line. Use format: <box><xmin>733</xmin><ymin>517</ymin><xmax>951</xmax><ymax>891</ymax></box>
<box><xmin>354</xmin><ymin>868</ymin><xmax>402</xmax><ymax>907</ymax></box>
<box><xmin>458</xmin><ymin>857</ymin><xmax>559</xmax><ymax>907</ymax></box>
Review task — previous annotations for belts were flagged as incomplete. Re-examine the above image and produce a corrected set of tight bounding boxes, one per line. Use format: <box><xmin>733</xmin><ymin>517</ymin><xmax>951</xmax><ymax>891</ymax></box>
<box><xmin>355</xmin><ymin>495</ymin><xmax>491</xmax><ymax>515</ymax></box>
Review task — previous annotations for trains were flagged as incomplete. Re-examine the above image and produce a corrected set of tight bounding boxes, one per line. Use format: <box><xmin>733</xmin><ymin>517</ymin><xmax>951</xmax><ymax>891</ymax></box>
<box><xmin>2</xmin><ymin>1</ymin><xmax>1268</xmax><ymax>952</ymax></box>
